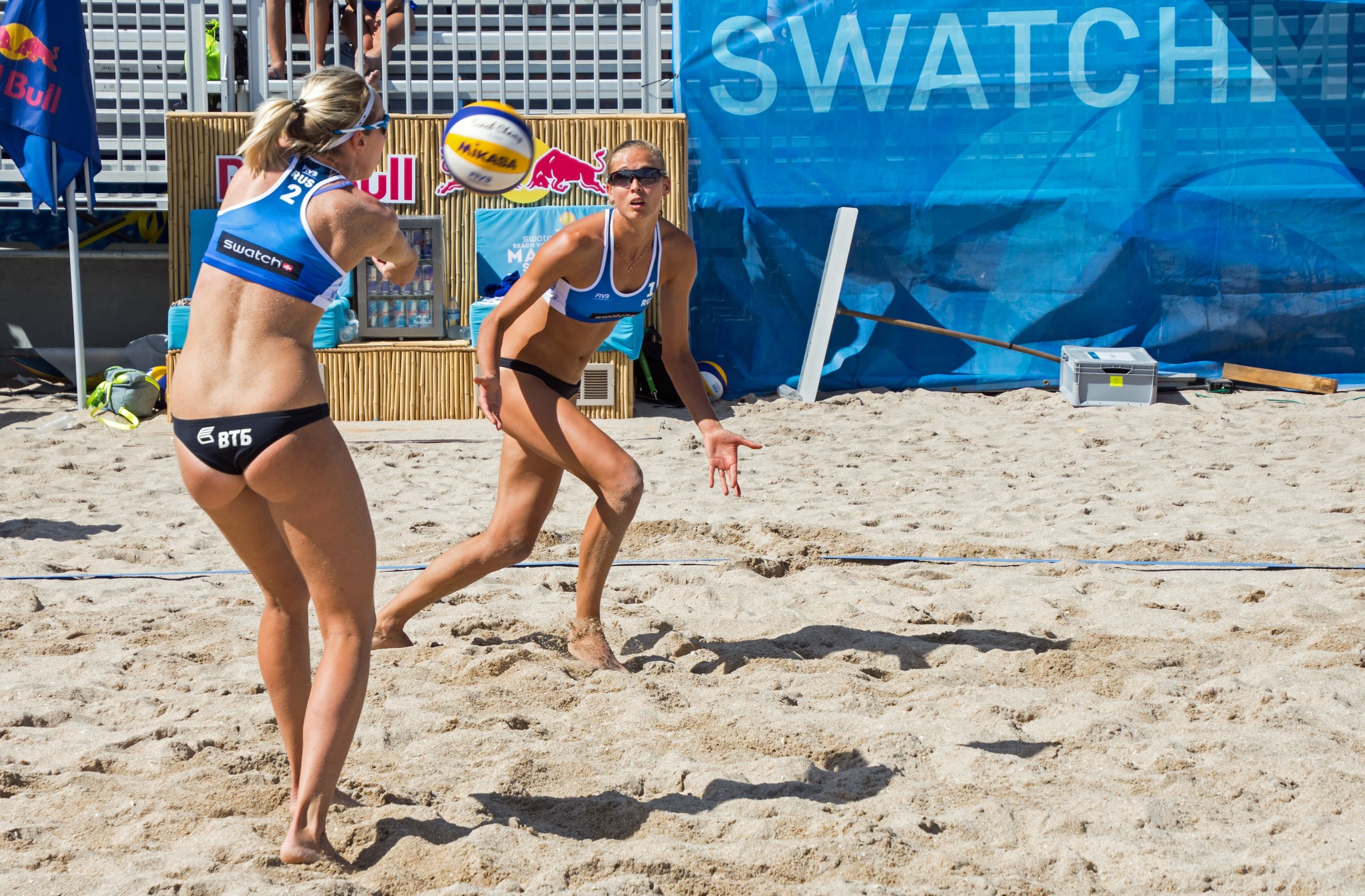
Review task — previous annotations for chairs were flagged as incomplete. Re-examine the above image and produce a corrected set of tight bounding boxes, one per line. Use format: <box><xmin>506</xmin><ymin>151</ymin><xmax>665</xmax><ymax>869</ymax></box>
<box><xmin>167</xmin><ymin>209</ymin><xmax>356</xmax><ymax>349</ymax></box>
<box><xmin>469</xmin><ymin>203</ymin><xmax>646</xmax><ymax>362</ymax></box>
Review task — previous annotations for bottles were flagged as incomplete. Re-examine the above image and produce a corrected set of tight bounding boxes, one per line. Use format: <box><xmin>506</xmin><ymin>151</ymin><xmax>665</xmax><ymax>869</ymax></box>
<box><xmin>38</xmin><ymin>415</ymin><xmax>77</xmax><ymax>436</ymax></box>
<box><xmin>447</xmin><ymin>325</ymin><xmax>471</xmax><ymax>340</ymax></box>
<box><xmin>447</xmin><ymin>296</ymin><xmax>461</xmax><ymax>327</ymax></box>
<box><xmin>777</xmin><ymin>384</ymin><xmax>799</xmax><ymax>401</ymax></box>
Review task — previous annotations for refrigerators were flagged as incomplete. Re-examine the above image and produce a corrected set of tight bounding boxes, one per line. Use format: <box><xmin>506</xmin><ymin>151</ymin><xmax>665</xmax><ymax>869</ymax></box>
<box><xmin>357</xmin><ymin>213</ymin><xmax>446</xmax><ymax>340</ymax></box>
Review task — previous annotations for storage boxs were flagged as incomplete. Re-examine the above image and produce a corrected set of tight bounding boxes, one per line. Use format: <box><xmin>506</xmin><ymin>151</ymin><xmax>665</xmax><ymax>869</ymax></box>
<box><xmin>1059</xmin><ymin>345</ymin><xmax>1158</xmax><ymax>407</ymax></box>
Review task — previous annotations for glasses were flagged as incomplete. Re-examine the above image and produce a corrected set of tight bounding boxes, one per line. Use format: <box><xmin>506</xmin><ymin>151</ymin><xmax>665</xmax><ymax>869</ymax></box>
<box><xmin>604</xmin><ymin>166</ymin><xmax>669</xmax><ymax>188</ymax></box>
<box><xmin>334</xmin><ymin>113</ymin><xmax>389</xmax><ymax>134</ymax></box>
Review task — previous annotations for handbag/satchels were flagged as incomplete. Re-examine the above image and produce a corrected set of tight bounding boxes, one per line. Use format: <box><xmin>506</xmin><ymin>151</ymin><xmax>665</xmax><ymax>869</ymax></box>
<box><xmin>324</xmin><ymin>43</ymin><xmax>355</xmax><ymax>70</ymax></box>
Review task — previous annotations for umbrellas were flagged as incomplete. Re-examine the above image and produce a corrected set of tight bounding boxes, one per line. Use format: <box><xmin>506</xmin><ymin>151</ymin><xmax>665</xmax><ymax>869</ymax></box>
<box><xmin>0</xmin><ymin>0</ymin><xmax>103</xmax><ymax>410</ymax></box>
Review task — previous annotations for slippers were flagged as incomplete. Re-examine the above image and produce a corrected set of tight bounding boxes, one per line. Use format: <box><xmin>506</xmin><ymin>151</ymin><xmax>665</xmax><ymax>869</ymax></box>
<box><xmin>267</xmin><ymin>68</ymin><xmax>281</xmax><ymax>80</ymax></box>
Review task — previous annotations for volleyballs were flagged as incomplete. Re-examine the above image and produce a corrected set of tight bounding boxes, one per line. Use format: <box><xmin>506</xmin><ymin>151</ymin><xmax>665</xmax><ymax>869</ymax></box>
<box><xmin>440</xmin><ymin>99</ymin><xmax>535</xmax><ymax>198</ymax></box>
<box><xmin>695</xmin><ymin>361</ymin><xmax>727</xmax><ymax>400</ymax></box>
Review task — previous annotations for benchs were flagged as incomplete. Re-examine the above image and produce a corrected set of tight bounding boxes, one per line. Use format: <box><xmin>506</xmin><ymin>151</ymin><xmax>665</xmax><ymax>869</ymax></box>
<box><xmin>0</xmin><ymin>0</ymin><xmax>674</xmax><ymax>212</ymax></box>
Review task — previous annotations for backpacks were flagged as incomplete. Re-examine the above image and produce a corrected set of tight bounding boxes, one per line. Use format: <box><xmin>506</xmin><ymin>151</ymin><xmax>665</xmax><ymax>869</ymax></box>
<box><xmin>633</xmin><ymin>323</ymin><xmax>684</xmax><ymax>408</ymax></box>
<box><xmin>182</xmin><ymin>19</ymin><xmax>248</xmax><ymax>112</ymax></box>
<box><xmin>86</xmin><ymin>365</ymin><xmax>160</xmax><ymax>429</ymax></box>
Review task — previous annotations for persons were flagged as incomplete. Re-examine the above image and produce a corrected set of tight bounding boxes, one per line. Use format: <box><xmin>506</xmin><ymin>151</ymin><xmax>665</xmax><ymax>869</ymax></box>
<box><xmin>372</xmin><ymin>141</ymin><xmax>763</xmax><ymax>674</ymax></box>
<box><xmin>265</xmin><ymin>0</ymin><xmax>341</xmax><ymax>81</ymax></box>
<box><xmin>341</xmin><ymin>0</ymin><xmax>417</xmax><ymax>89</ymax></box>
<box><xmin>172</xmin><ymin>66</ymin><xmax>420</xmax><ymax>875</ymax></box>
